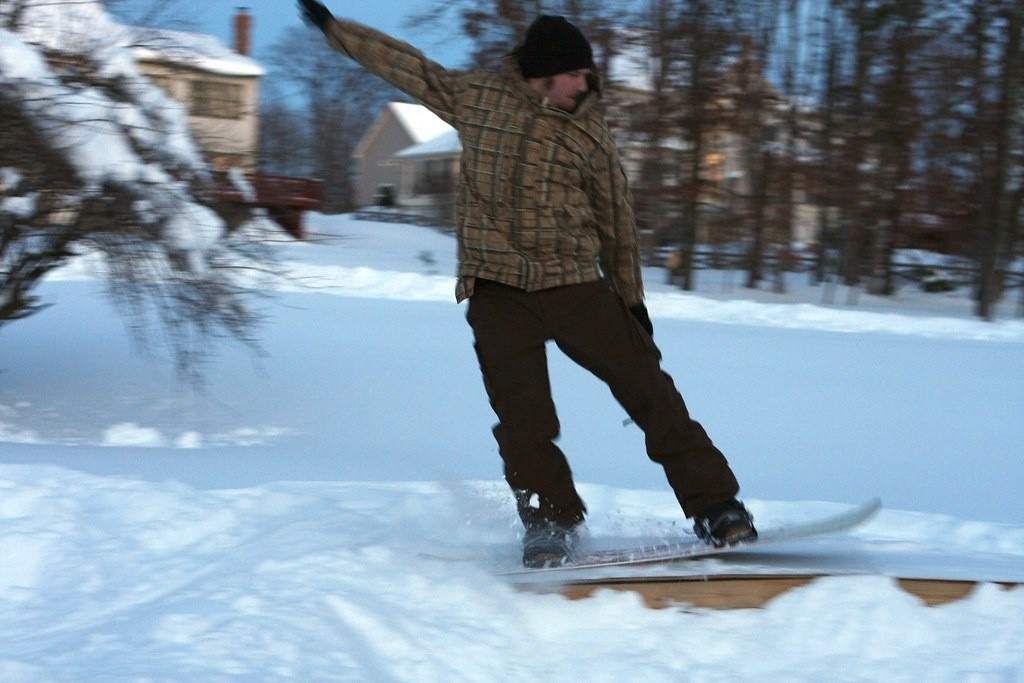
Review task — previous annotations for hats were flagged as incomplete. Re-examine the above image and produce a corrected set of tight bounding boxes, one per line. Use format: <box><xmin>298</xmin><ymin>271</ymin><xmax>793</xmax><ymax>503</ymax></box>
<box><xmin>517</xmin><ymin>15</ymin><xmax>593</xmax><ymax>79</ymax></box>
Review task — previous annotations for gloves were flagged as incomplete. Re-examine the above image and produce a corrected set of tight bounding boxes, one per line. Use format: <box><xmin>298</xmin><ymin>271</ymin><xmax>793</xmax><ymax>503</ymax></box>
<box><xmin>632</xmin><ymin>304</ymin><xmax>654</xmax><ymax>338</ymax></box>
<box><xmin>296</xmin><ymin>1</ymin><xmax>334</xmax><ymax>35</ymax></box>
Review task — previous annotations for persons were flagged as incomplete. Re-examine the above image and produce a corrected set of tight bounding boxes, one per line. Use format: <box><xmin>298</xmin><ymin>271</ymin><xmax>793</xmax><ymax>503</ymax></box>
<box><xmin>292</xmin><ymin>0</ymin><xmax>758</xmax><ymax>567</ymax></box>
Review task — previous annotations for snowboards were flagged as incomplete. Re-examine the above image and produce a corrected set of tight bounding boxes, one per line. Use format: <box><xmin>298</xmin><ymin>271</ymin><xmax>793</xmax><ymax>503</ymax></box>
<box><xmin>421</xmin><ymin>493</ymin><xmax>885</xmax><ymax>579</ymax></box>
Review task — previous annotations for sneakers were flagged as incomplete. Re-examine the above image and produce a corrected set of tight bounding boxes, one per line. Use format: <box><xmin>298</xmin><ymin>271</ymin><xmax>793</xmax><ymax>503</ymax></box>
<box><xmin>693</xmin><ymin>497</ymin><xmax>758</xmax><ymax>548</ymax></box>
<box><xmin>522</xmin><ymin>510</ymin><xmax>586</xmax><ymax>569</ymax></box>
